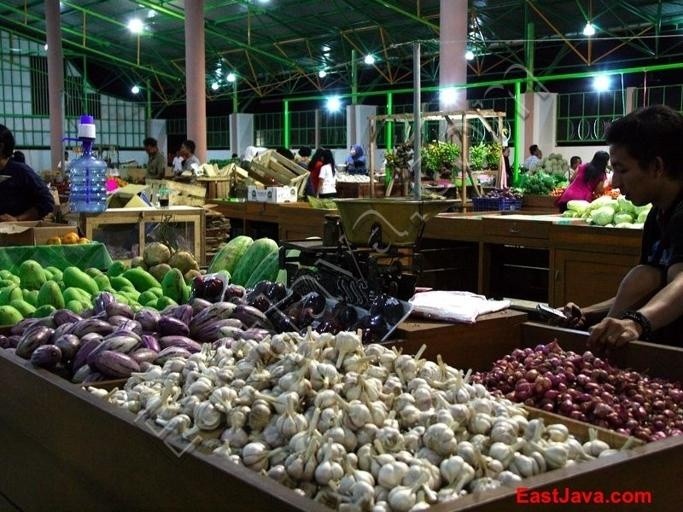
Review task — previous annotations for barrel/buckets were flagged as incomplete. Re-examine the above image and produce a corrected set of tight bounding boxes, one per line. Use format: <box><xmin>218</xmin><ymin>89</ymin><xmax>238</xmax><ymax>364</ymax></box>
<box><xmin>59</xmin><ymin>117</ymin><xmax>107</xmax><ymax>214</ymax></box>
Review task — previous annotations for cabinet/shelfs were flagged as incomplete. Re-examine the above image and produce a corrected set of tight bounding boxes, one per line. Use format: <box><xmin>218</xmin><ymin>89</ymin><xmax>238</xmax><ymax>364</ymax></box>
<box><xmin>210</xmin><ymin>196</ymin><xmax>343</xmax><ymax>245</ymax></box>
<box><xmin>2</xmin><ymin>263</ymin><xmax>683</xmax><ymax>511</ymax></box>
<box><xmin>420</xmin><ymin>207</ymin><xmax>668</xmax><ymax>331</ymax></box>
<box><xmin>79</xmin><ymin>206</ymin><xmax>206</xmax><ymax>272</ymax></box>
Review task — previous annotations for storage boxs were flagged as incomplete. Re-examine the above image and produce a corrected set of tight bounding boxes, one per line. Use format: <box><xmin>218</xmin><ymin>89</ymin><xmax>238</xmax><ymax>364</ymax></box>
<box><xmin>0</xmin><ymin>221</ymin><xmax>78</xmax><ymax>246</ymax></box>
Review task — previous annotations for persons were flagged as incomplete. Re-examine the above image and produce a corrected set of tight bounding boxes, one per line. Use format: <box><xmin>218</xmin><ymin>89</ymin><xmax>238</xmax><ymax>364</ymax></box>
<box><xmin>317</xmin><ymin>149</ymin><xmax>338</xmax><ymax>199</ymax></box>
<box><xmin>565</xmin><ymin>157</ymin><xmax>582</xmax><ymax>182</ymax></box>
<box><xmin>344</xmin><ymin>144</ymin><xmax>368</xmax><ymax>175</ymax></box>
<box><xmin>307</xmin><ymin>149</ymin><xmax>324</xmax><ymax>198</ymax></box>
<box><xmin>557</xmin><ymin>151</ymin><xmax>610</xmax><ymax>215</ymax></box>
<box><xmin>545</xmin><ymin>104</ymin><xmax>683</xmax><ymax>358</ymax></box>
<box><xmin>503</xmin><ymin>147</ymin><xmax>513</xmax><ymax>188</ymax></box>
<box><xmin>0</xmin><ymin>123</ymin><xmax>56</xmax><ymax>223</ymax></box>
<box><xmin>180</xmin><ymin>140</ymin><xmax>203</xmax><ymax>179</ymax></box>
<box><xmin>137</xmin><ymin>137</ymin><xmax>165</xmax><ymax>185</ymax></box>
<box><xmin>299</xmin><ymin>145</ymin><xmax>313</xmax><ymax>167</ymax></box>
<box><xmin>522</xmin><ymin>145</ymin><xmax>543</xmax><ymax>174</ymax></box>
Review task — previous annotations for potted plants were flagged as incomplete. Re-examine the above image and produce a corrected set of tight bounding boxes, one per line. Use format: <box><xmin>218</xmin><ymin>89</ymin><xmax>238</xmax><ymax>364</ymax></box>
<box><xmin>420</xmin><ymin>144</ymin><xmax>438</xmax><ymax>179</ymax></box>
<box><xmin>485</xmin><ymin>144</ymin><xmax>501</xmax><ymax>172</ymax></box>
<box><xmin>469</xmin><ymin>142</ymin><xmax>485</xmax><ymax>171</ymax></box>
<box><xmin>436</xmin><ymin>140</ymin><xmax>462</xmax><ymax>178</ymax></box>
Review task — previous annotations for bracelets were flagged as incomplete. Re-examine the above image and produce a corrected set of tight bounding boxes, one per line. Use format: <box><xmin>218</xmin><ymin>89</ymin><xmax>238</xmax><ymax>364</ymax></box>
<box><xmin>622</xmin><ymin>309</ymin><xmax>652</xmax><ymax>343</ymax></box>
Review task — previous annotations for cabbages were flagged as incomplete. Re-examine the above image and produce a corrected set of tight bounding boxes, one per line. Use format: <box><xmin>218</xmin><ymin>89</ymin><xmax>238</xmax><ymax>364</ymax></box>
<box><xmin>562</xmin><ymin>194</ymin><xmax>652</xmax><ymax>228</ymax></box>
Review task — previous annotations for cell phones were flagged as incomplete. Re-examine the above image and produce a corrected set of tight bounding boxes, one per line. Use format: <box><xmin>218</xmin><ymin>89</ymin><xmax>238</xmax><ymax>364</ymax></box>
<box><xmin>534</xmin><ymin>304</ymin><xmax>569</xmax><ymax>320</ymax></box>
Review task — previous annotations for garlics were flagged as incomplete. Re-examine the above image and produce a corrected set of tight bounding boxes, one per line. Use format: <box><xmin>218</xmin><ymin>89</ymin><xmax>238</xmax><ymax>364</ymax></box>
<box><xmin>82</xmin><ymin>325</ymin><xmax>634</xmax><ymax>512</ymax></box>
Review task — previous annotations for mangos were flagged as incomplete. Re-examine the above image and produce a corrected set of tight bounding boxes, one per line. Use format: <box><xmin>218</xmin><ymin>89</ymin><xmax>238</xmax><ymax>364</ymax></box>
<box><xmin>0</xmin><ymin>259</ymin><xmax>190</xmax><ymax>326</ymax></box>
